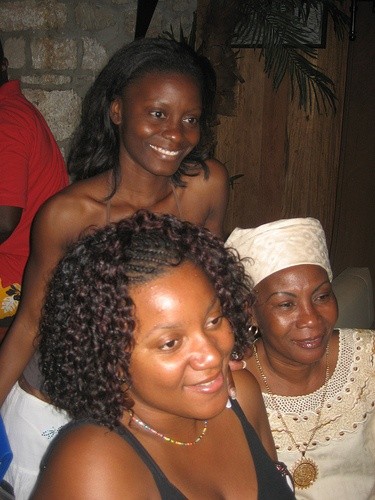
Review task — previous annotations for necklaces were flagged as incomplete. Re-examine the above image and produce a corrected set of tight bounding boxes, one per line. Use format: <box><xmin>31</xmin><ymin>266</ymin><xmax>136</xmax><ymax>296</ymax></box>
<box><xmin>253</xmin><ymin>337</ymin><xmax>329</xmax><ymax>490</ymax></box>
<box><xmin>128</xmin><ymin>410</ymin><xmax>208</xmax><ymax>447</ymax></box>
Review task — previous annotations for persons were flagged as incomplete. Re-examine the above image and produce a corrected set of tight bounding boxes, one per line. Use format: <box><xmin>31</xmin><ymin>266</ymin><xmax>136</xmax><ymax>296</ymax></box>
<box><xmin>0</xmin><ymin>40</ymin><xmax>70</xmax><ymax>347</ymax></box>
<box><xmin>0</xmin><ymin>40</ymin><xmax>231</xmax><ymax>500</ymax></box>
<box><xmin>27</xmin><ymin>208</ymin><xmax>296</xmax><ymax>500</ymax></box>
<box><xmin>224</xmin><ymin>216</ymin><xmax>375</xmax><ymax>500</ymax></box>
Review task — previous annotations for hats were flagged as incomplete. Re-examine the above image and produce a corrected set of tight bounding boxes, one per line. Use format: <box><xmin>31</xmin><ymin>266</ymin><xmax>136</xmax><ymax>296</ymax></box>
<box><xmin>223</xmin><ymin>217</ymin><xmax>333</xmax><ymax>290</ymax></box>
